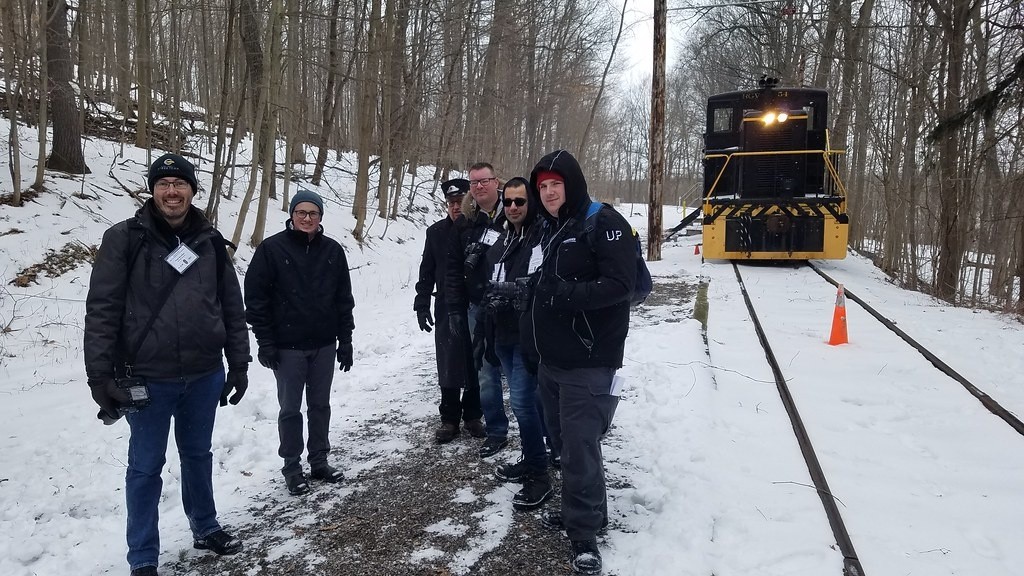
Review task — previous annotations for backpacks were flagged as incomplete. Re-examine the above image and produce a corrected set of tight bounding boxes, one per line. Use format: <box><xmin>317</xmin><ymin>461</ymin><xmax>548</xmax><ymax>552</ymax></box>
<box><xmin>542</xmin><ymin>202</ymin><xmax>653</xmax><ymax>307</ymax></box>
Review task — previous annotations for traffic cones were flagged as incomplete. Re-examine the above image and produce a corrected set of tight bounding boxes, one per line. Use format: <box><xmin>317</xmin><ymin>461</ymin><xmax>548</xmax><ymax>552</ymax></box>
<box><xmin>694</xmin><ymin>245</ymin><xmax>700</xmax><ymax>255</ymax></box>
<box><xmin>825</xmin><ymin>284</ymin><xmax>849</xmax><ymax>346</ymax></box>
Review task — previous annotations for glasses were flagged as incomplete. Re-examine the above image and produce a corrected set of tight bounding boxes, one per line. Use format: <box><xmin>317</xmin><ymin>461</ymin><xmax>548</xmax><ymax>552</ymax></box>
<box><xmin>155</xmin><ymin>178</ymin><xmax>189</xmax><ymax>190</ymax></box>
<box><xmin>293</xmin><ymin>209</ymin><xmax>322</xmax><ymax>220</ymax></box>
<box><xmin>468</xmin><ymin>177</ymin><xmax>496</xmax><ymax>187</ymax></box>
<box><xmin>502</xmin><ymin>197</ymin><xmax>529</xmax><ymax>207</ymax></box>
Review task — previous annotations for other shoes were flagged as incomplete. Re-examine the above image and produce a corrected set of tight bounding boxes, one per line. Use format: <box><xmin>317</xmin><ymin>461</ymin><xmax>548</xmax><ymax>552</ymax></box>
<box><xmin>286</xmin><ymin>472</ymin><xmax>310</xmax><ymax>496</ymax></box>
<box><xmin>311</xmin><ymin>465</ymin><xmax>345</xmax><ymax>483</ymax></box>
<box><xmin>194</xmin><ymin>530</ymin><xmax>243</xmax><ymax>555</ymax></box>
<box><xmin>131</xmin><ymin>563</ymin><xmax>158</xmax><ymax>576</ymax></box>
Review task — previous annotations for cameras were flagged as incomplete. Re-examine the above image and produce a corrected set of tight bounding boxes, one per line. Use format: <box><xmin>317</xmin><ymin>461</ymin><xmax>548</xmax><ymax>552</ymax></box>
<box><xmin>462</xmin><ymin>241</ymin><xmax>485</xmax><ymax>268</ymax></box>
<box><xmin>97</xmin><ymin>375</ymin><xmax>153</xmax><ymax>426</ymax></box>
<box><xmin>487</xmin><ymin>296</ymin><xmax>511</xmax><ymax>314</ymax></box>
<box><xmin>488</xmin><ymin>275</ymin><xmax>543</xmax><ymax>313</ymax></box>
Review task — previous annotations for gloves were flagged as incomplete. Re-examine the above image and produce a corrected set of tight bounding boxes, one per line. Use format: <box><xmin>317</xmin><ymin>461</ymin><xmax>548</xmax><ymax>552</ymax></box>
<box><xmin>87</xmin><ymin>376</ymin><xmax>131</xmax><ymax>419</ymax></box>
<box><xmin>258</xmin><ymin>339</ymin><xmax>281</xmax><ymax>370</ymax></box>
<box><xmin>336</xmin><ymin>336</ymin><xmax>353</xmax><ymax>372</ymax></box>
<box><xmin>472</xmin><ymin>336</ymin><xmax>485</xmax><ymax>371</ymax></box>
<box><xmin>448</xmin><ymin>311</ymin><xmax>465</xmax><ymax>337</ymax></box>
<box><xmin>417</xmin><ymin>306</ymin><xmax>435</xmax><ymax>333</ymax></box>
<box><xmin>219</xmin><ymin>362</ymin><xmax>248</xmax><ymax>405</ymax></box>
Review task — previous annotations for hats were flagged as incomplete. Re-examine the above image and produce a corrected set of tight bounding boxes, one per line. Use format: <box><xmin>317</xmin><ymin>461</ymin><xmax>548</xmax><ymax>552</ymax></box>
<box><xmin>441</xmin><ymin>179</ymin><xmax>470</xmax><ymax>202</ymax></box>
<box><xmin>290</xmin><ymin>191</ymin><xmax>324</xmax><ymax>219</ymax></box>
<box><xmin>148</xmin><ymin>154</ymin><xmax>198</xmax><ymax>200</ymax></box>
<box><xmin>536</xmin><ymin>169</ymin><xmax>564</xmax><ymax>190</ymax></box>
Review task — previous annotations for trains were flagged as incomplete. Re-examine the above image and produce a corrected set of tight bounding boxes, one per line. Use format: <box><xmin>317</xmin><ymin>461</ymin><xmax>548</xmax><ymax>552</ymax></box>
<box><xmin>700</xmin><ymin>73</ymin><xmax>850</xmax><ymax>266</ymax></box>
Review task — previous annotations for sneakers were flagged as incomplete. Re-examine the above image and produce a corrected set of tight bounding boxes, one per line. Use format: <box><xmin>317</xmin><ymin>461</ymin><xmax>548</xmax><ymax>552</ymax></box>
<box><xmin>436</xmin><ymin>421</ymin><xmax>460</xmax><ymax>441</ymax></box>
<box><xmin>570</xmin><ymin>538</ymin><xmax>602</xmax><ymax>573</ymax></box>
<box><xmin>540</xmin><ymin>508</ymin><xmax>609</xmax><ymax>530</ymax></box>
<box><xmin>464</xmin><ymin>420</ymin><xmax>487</xmax><ymax>438</ymax></box>
<box><xmin>496</xmin><ymin>435</ymin><xmax>564</xmax><ymax>510</ymax></box>
<box><xmin>480</xmin><ymin>436</ymin><xmax>508</xmax><ymax>456</ymax></box>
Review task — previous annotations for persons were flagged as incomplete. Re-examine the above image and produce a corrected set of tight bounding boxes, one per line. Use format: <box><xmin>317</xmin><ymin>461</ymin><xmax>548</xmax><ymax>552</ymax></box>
<box><xmin>84</xmin><ymin>154</ymin><xmax>253</xmax><ymax>576</ymax></box>
<box><xmin>520</xmin><ymin>149</ymin><xmax>635</xmax><ymax>574</ymax></box>
<box><xmin>413</xmin><ymin>162</ymin><xmax>560</xmax><ymax>510</ymax></box>
<box><xmin>245</xmin><ymin>190</ymin><xmax>355</xmax><ymax>496</ymax></box>
